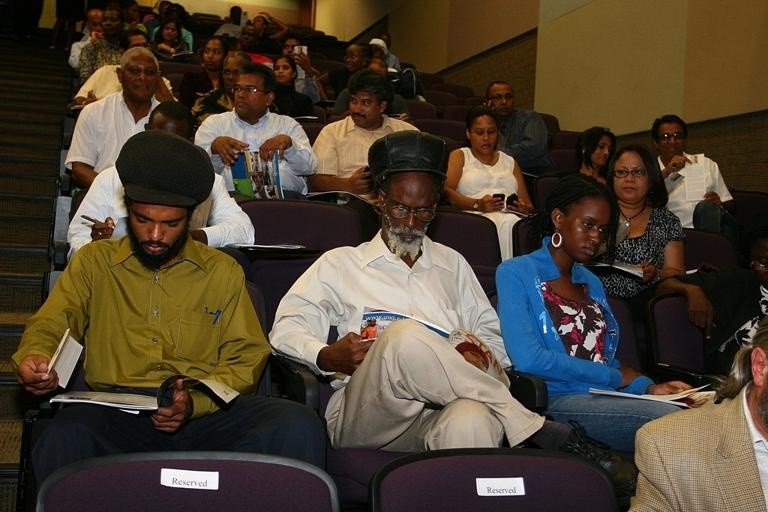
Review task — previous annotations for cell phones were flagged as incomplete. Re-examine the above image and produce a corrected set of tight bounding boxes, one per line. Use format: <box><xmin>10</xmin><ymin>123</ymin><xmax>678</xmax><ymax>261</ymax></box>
<box><xmin>493</xmin><ymin>194</ymin><xmax>505</xmax><ymax>201</ymax></box>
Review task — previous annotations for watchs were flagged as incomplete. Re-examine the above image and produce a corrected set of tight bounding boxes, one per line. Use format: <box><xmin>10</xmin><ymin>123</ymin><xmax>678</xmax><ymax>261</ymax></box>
<box><xmin>473</xmin><ymin>200</ymin><xmax>479</xmax><ymax>209</ymax></box>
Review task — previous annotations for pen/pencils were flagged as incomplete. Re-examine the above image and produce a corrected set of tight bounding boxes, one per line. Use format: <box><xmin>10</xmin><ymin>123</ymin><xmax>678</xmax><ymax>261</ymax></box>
<box><xmin>81</xmin><ymin>215</ymin><xmax>114</xmax><ymax>230</ymax></box>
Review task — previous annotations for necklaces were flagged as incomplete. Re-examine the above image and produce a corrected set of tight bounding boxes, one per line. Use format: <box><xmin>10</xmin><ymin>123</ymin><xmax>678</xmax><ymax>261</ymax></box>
<box><xmin>620</xmin><ymin>201</ymin><xmax>647</xmax><ymax>227</ymax></box>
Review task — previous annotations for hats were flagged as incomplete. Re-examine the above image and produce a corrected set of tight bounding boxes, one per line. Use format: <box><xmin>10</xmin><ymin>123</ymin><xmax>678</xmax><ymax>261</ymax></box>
<box><xmin>367</xmin><ymin>130</ymin><xmax>451</xmax><ymax>182</ymax></box>
<box><xmin>369</xmin><ymin>38</ymin><xmax>387</xmax><ymax>54</ymax></box>
<box><xmin>115</xmin><ymin>130</ymin><xmax>215</xmax><ymax>209</ymax></box>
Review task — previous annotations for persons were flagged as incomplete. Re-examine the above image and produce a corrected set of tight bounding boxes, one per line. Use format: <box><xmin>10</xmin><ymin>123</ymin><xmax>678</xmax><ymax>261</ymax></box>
<box><xmin>597</xmin><ymin>143</ymin><xmax>687</xmax><ymax>308</ymax></box>
<box><xmin>66</xmin><ymin>101</ymin><xmax>255</xmax><ymax>266</ymax></box>
<box><xmin>56</xmin><ymin>0</ymin><xmax>418</xmax><ymax>125</ymax></box>
<box><xmin>456</xmin><ymin>342</ymin><xmax>489</xmax><ymax>372</ymax></box>
<box><xmin>487</xmin><ymin>81</ymin><xmax>554</xmax><ymax>171</ymax></box>
<box><xmin>195</xmin><ymin>63</ymin><xmax>318</xmax><ymax>195</ymax></box>
<box><xmin>576</xmin><ymin>126</ymin><xmax>616</xmax><ymax>187</ymax></box>
<box><xmin>269</xmin><ymin>130</ymin><xmax>636</xmax><ymax>492</ymax></box>
<box><xmin>13</xmin><ymin>130</ymin><xmax>327</xmax><ymax>489</ymax></box>
<box><xmin>496</xmin><ymin>177</ymin><xmax>693</xmax><ymax>449</ymax></box>
<box><xmin>649</xmin><ymin>222</ymin><xmax>768</xmax><ymax>376</ymax></box>
<box><xmin>629</xmin><ymin>317</ymin><xmax>768</xmax><ymax>512</ymax></box>
<box><xmin>305</xmin><ymin>71</ymin><xmax>418</xmax><ymax>213</ymax></box>
<box><xmin>444</xmin><ymin>110</ymin><xmax>534</xmax><ymax>261</ymax></box>
<box><xmin>362</xmin><ymin>319</ymin><xmax>377</xmax><ymax>339</ymax></box>
<box><xmin>64</xmin><ymin>46</ymin><xmax>159</xmax><ymax>221</ymax></box>
<box><xmin>652</xmin><ymin>114</ymin><xmax>733</xmax><ymax>231</ymax></box>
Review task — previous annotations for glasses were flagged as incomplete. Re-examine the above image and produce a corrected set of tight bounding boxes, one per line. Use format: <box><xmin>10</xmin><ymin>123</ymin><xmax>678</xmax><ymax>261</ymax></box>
<box><xmin>657</xmin><ymin>133</ymin><xmax>683</xmax><ymax>140</ymax></box>
<box><xmin>381</xmin><ymin>195</ymin><xmax>436</xmax><ymax>223</ymax></box>
<box><xmin>231</xmin><ymin>85</ymin><xmax>268</xmax><ymax>94</ymax></box>
<box><xmin>612</xmin><ymin>169</ymin><xmax>648</xmax><ymax>178</ymax></box>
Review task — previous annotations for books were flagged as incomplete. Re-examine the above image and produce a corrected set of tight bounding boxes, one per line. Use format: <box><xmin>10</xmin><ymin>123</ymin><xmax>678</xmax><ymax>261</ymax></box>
<box><xmin>213</xmin><ymin>149</ymin><xmax>284</xmax><ymax>199</ymax></box>
<box><xmin>360</xmin><ymin>305</ymin><xmax>511</xmax><ymax>388</ymax></box>
<box><xmin>590</xmin><ymin>383</ymin><xmax>711</xmax><ymax>408</ymax></box>
<box><xmin>588</xmin><ymin>260</ymin><xmax>643</xmax><ymax>278</ymax></box>
<box><xmin>50</xmin><ymin>375</ymin><xmax>239</xmax><ymax>410</ymax></box>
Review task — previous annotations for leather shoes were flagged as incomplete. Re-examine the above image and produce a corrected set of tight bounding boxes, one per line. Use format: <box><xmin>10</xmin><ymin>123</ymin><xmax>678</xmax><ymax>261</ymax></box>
<box><xmin>563</xmin><ymin>420</ymin><xmax>637</xmax><ymax>494</ymax></box>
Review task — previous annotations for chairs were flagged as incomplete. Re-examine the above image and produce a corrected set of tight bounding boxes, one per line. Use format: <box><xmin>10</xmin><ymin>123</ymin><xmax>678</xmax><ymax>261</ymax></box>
<box><xmin>16</xmin><ymin>2</ymin><xmax>768</xmax><ymax>511</ymax></box>
